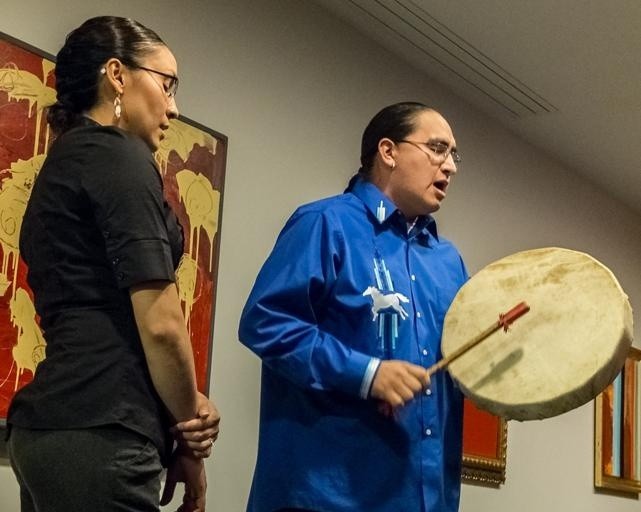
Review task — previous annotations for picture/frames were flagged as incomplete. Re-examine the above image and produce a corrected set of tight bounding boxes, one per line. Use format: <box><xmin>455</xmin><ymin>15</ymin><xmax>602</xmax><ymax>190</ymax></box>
<box><xmin>0</xmin><ymin>31</ymin><xmax>230</xmax><ymax>430</ymax></box>
<box><xmin>594</xmin><ymin>343</ymin><xmax>641</xmax><ymax>495</ymax></box>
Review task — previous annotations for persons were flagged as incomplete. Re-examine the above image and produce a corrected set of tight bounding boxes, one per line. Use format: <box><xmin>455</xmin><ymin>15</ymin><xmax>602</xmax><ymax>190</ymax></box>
<box><xmin>4</xmin><ymin>15</ymin><xmax>220</xmax><ymax>512</ymax></box>
<box><xmin>238</xmin><ymin>102</ymin><xmax>471</xmax><ymax>512</ymax></box>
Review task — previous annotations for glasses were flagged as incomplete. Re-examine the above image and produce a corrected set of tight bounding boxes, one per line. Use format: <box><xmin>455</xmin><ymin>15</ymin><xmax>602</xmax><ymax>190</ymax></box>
<box><xmin>393</xmin><ymin>140</ymin><xmax>465</xmax><ymax>169</ymax></box>
<box><xmin>120</xmin><ymin>60</ymin><xmax>180</xmax><ymax>97</ymax></box>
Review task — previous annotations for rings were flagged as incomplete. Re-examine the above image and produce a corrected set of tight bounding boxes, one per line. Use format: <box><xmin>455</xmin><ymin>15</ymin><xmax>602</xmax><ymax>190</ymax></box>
<box><xmin>208</xmin><ymin>438</ymin><xmax>215</xmax><ymax>448</ymax></box>
